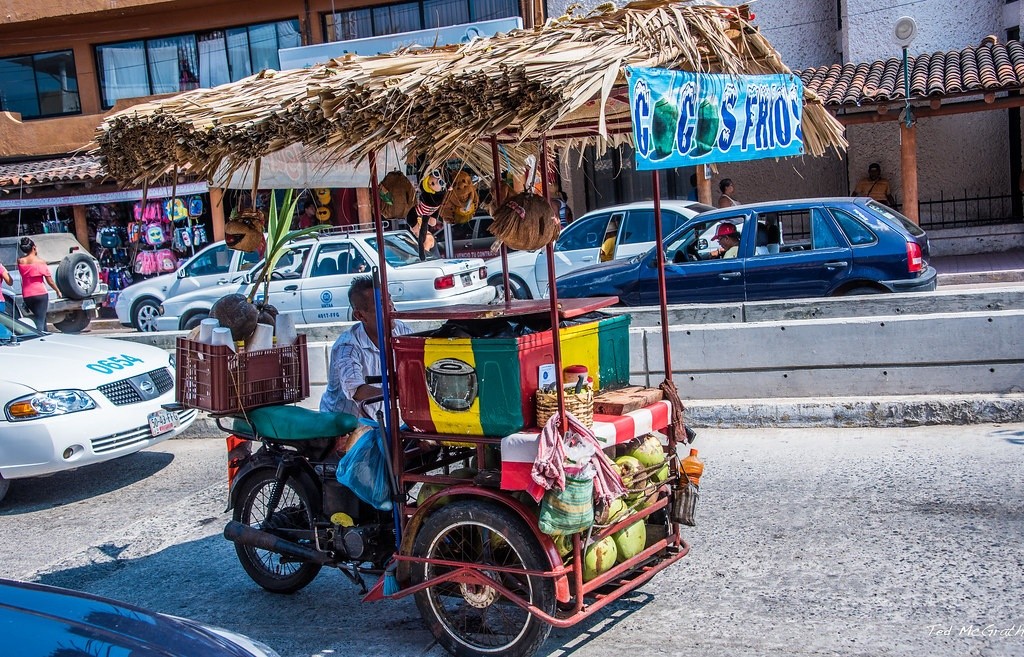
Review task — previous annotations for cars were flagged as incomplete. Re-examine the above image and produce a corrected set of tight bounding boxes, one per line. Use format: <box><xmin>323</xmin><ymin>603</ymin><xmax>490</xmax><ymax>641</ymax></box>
<box><xmin>485</xmin><ymin>200</ymin><xmax>783</xmax><ymax>300</ymax></box>
<box><xmin>115</xmin><ymin>221</ymin><xmax>496</xmax><ymax>332</ymax></box>
<box><xmin>432</xmin><ymin>216</ymin><xmax>512</xmax><ymax>259</ymax></box>
<box><xmin>0</xmin><ymin>312</ymin><xmax>198</xmax><ymax>503</ymax></box>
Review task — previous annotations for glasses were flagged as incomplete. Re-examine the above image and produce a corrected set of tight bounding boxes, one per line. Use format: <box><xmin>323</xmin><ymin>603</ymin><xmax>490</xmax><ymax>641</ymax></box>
<box><xmin>717</xmin><ymin>237</ymin><xmax>724</xmax><ymax>243</ymax></box>
<box><xmin>731</xmin><ymin>184</ymin><xmax>735</xmax><ymax>186</ymax></box>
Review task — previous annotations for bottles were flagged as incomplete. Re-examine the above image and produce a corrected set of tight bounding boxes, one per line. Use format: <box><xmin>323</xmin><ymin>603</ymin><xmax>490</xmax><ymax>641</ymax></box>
<box><xmin>236</xmin><ymin>340</ymin><xmax>245</xmax><ymax>353</ymax></box>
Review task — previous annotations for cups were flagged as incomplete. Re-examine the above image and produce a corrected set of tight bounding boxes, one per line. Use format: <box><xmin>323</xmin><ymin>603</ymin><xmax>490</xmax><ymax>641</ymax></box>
<box><xmin>199</xmin><ymin>318</ymin><xmax>220</xmax><ymax>343</ymax></box>
<box><xmin>211</xmin><ymin>326</ymin><xmax>235</xmax><ymax>352</ymax></box>
<box><xmin>562</xmin><ymin>364</ymin><xmax>588</xmax><ymax>383</ymax></box>
<box><xmin>247</xmin><ymin>323</ymin><xmax>274</xmax><ymax>352</ymax></box>
<box><xmin>276</xmin><ymin>313</ymin><xmax>297</xmax><ymax>349</ymax></box>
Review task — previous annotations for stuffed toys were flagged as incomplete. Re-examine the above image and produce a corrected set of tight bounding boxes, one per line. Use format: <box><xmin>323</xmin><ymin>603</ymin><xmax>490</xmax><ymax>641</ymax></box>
<box><xmin>406</xmin><ymin>151</ymin><xmax>453</xmax><ymax>262</ymax></box>
<box><xmin>441</xmin><ymin>172</ymin><xmax>478</xmax><ymax>225</ymax></box>
<box><xmin>314</xmin><ymin>188</ymin><xmax>331</xmax><ymax>220</ymax></box>
<box><xmin>225</xmin><ymin>210</ymin><xmax>264</xmax><ymax>253</ymax></box>
<box><xmin>480</xmin><ymin>168</ymin><xmax>542</xmax><ymax>216</ymax></box>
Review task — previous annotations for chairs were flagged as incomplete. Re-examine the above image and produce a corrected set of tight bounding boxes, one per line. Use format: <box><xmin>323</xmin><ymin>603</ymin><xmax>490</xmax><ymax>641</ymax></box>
<box><xmin>314</xmin><ymin>258</ymin><xmax>337</xmax><ymax>276</ymax></box>
<box><xmin>755</xmin><ymin>223</ymin><xmax>770</xmax><ymax>257</ymax></box>
<box><xmin>338</xmin><ymin>251</ymin><xmax>355</xmax><ymax>273</ymax></box>
<box><xmin>764</xmin><ymin>225</ymin><xmax>783</xmax><ymax>254</ymax></box>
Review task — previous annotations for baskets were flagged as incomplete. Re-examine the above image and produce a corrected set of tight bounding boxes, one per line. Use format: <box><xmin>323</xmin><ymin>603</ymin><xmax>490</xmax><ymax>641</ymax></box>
<box><xmin>535</xmin><ymin>385</ymin><xmax>594</xmax><ymax>433</ymax></box>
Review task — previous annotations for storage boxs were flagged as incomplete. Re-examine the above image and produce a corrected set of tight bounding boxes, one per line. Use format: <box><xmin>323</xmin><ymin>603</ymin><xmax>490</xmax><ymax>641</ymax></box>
<box><xmin>389</xmin><ymin>297</ymin><xmax>630</xmax><ymax>435</ymax></box>
<box><xmin>172</xmin><ymin>333</ymin><xmax>309</xmax><ymax>413</ymax></box>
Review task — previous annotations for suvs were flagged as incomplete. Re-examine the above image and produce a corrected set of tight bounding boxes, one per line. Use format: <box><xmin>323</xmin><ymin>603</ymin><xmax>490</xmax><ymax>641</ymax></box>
<box><xmin>542</xmin><ymin>197</ymin><xmax>937</xmax><ymax>307</ymax></box>
<box><xmin>0</xmin><ymin>233</ymin><xmax>108</xmax><ymax>333</ymax></box>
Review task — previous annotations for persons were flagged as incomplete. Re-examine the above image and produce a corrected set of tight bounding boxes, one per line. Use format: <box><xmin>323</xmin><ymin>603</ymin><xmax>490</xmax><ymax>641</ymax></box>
<box><xmin>718</xmin><ymin>179</ymin><xmax>744</xmax><ymax>224</ymax></box>
<box><xmin>600</xmin><ymin>222</ymin><xmax>617</xmax><ymax>262</ymax></box>
<box><xmin>710</xmin><ymin>221</ymin><xmax>740</xmax><ymax>259</ymax></box>
<box><xmin>687</xmin><ymin>174</ymin><xmax>698</xmax><ymax>202</ymax></box>
<box><xmin>851</xmin><ymin>163</ymin><xmax>899</xmax><ymax>211</ymax></box>
<box><xmin>298</xmin><ymin>202</ymin><xmax>316</xmax><ymax>229</ymax></box>
<box><xmin>321</xmin><ymin>277</ymin><xmax>441</xmax><ymax>570</ymax></box>
<box><xmin>17</xmin><ymin>237</ymin><xmax>62</xmax><ymax>332</ymax></box>
<box><xmin>0</xmin><ymin>263</ymin><xmax>14</xmax><ymax>334</ymax></box>
<box><xmin>555</xmin><ymin>191</ymin><xmax>568</xmax><ymax>229</ymax></box>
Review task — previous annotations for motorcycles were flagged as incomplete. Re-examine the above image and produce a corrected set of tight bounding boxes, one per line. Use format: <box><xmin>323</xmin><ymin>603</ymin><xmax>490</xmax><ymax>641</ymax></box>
<box><xmin>95</xmin><ymin>1</ymin><xmax>842</xmax><ymax>657</ymax></box>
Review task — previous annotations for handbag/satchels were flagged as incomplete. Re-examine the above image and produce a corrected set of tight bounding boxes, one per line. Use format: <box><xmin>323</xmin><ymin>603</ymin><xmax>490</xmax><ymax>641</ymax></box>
<box><xmin>335</xmin><ymin>416</ymin><xmax>409</xmax><ymax>511</ymax></box>
<box><xmin>538</xmin><ymin>446</ymin><xmax>595</xmax><ymax>536</ymax></box>
<box><xmin>672</xmin><ymin>481</ymin><xmax>700</xmax><ymax>527</ymax></box>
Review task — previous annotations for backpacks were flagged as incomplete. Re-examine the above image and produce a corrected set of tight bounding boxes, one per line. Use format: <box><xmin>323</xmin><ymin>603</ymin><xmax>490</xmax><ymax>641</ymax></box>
<box><xmin>86</xmin><ymin>197</ymin><xmax>207</xmax><ymax>308</ymax></box>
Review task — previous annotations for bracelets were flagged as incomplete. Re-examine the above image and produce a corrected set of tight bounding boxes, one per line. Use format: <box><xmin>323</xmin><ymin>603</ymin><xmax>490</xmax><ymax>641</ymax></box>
<box><xmin>717</xmin><ymin>249</ymin><xmax>720</xmax><ymax>254</ymax></box>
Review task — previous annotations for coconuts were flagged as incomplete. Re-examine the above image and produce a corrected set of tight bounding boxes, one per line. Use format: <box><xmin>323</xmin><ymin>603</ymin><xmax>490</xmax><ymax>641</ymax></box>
<box><xmin>208</xmin><ymin>293</ymin><xmax>277</xmax><ymax>342</ymax></box>
<box><xmin>416</xmin><ymin>432</ymin><xmax>669</xmax><ymax>585</ymax></box>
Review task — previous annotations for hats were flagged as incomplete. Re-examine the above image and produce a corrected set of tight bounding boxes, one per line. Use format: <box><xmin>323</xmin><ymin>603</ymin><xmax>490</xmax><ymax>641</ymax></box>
<box><xmin>607</xmin><ymin>221</ymin><xmax>618</xmax><ymax>232</ymax></box>
<box><xmin>869</xmin><ymin>163</ymin><xmax>880</xmax><ymax>171</ymax></box>
<box><xmin>711</xmin><ymin>222</ymin><xmax>737</xmax><ymax>241</ymax></box>
